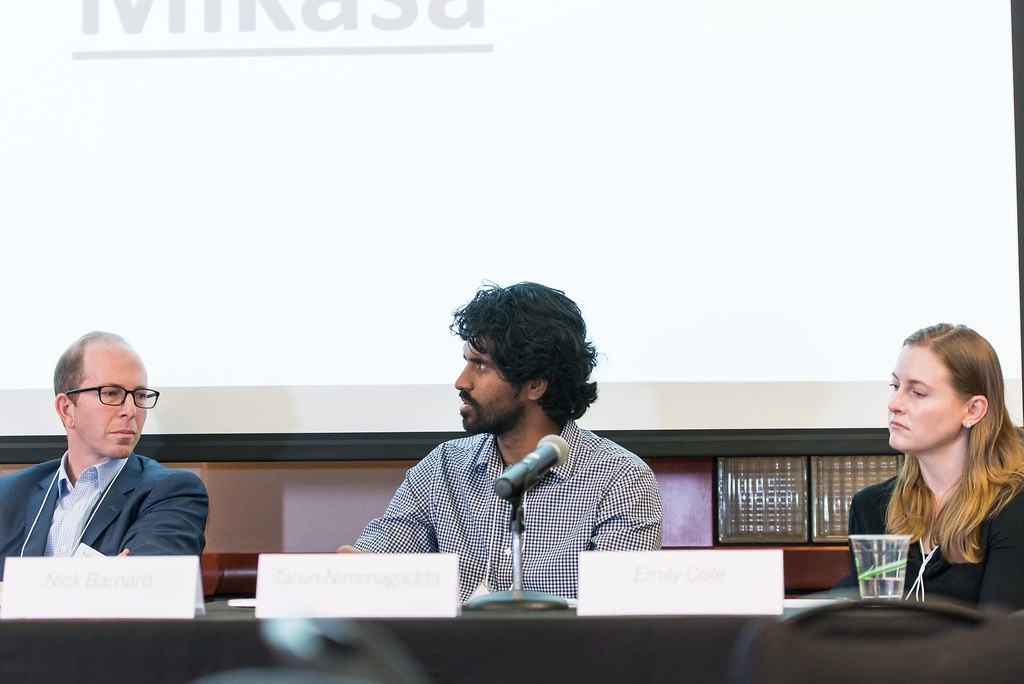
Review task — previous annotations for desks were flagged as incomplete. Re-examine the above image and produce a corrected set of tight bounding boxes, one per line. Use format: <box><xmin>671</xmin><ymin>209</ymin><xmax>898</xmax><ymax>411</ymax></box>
<box><xmin>0</xmin><ymin>601</ymin><xmax>806</xmax><ymax>684</ymax></box>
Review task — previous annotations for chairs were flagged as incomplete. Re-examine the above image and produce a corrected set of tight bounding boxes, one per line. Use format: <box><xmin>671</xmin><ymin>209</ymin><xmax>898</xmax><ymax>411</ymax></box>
<box><xmin>735</xmin><ymin>597</ymin><xmax>1024</xmax><ymax>684</ymax></box>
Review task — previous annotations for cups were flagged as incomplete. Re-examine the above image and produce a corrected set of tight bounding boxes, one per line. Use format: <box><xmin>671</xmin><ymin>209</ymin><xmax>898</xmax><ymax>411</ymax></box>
<box><xmin>849</xmin><ymin>534</ymin><xmax>910</xmax><ymax>607</ymax></box>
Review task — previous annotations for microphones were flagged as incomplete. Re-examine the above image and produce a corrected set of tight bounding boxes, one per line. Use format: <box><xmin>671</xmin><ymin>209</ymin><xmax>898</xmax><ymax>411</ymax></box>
<box><xmin>494</xmin><ymin>434</ymin><xmax>570</xmax><ymax>499</ymax></box>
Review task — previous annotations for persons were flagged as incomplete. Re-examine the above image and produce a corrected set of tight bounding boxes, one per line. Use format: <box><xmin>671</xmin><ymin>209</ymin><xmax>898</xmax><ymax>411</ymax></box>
<box><xmin>848</xmin><ymin>323</ymin><xmax>1024</xmax><ymax>617</ymax></box>
<box><xmin>0</xmin><ymin>330</ymin><xmax>209</xmax><ymax>581</ymax></box>
<box><xmin>329</xmin><ymin>280</ymin><xmax>662</xmax><ymax>599</ymax></box>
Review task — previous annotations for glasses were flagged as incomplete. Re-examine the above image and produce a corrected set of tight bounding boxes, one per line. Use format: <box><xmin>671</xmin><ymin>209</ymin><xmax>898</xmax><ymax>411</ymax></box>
<box><xmin>65</xmin><ymin>385</ymin><xmax>160</xmax><ymax>410</ymax></box>
